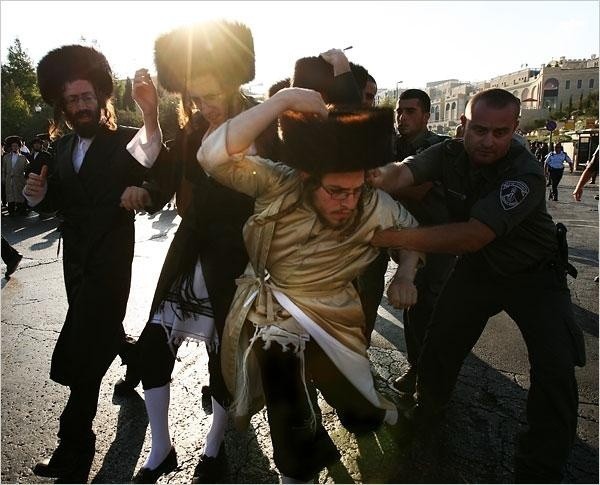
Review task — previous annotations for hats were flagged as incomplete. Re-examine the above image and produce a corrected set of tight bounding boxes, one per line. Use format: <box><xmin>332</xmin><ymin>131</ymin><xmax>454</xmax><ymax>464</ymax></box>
<box><xmin>5</xmin><ymin>133</ymin><xmax>49</xmax><ymax>145</ymax></box>
<box><xmin>153</xmin><ymin>22</ymin><xmax>255</xmax><ymax>93</ymax></box>
<box><xmin>37</xmin><ymin>46</ymin><xmax>113</xmax><ymax>107</ymax></box>
<box><xmin>269</xmin><ymin>56</ymin><xmax>396</xmax><ymax>172</ymax></box>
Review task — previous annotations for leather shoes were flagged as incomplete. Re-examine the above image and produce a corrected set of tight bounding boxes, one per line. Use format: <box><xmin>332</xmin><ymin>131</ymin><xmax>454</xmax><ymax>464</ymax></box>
<box><xmin>394</xmin><ymin>365</ymin><xmax>415</xmax><ymax>392</ymax></box>
<box><xmin>549</xmin><ymin>192</ymin><xmax>558</xmax><ymax>201</ymax></box>
<box><xmin>192</xmin><ymin>442</ymin><xmax>224</xmax><ymax>484</ymax></box>
<box><xmin>125</xmin><ymin>341</ymin><xmax>140</xmax><ymax>389</ymax></box>
<box><xmin>132</xmin><ymin>446</ymin><xmax>177</xmax><ymax>484</ymax></box>
<box><xmin>5</xmin><ymin>252</ymin><xmax>23</xmax><ymax>278</ymax></box>
<box><xmin>34</xmin><ymin>457</ymin><xmax>93</xmax><ymax>477</ymax></box>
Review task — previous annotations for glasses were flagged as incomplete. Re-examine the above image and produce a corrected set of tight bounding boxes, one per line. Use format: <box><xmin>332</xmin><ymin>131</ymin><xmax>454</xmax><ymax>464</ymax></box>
<box><xmin>320</xmin><ymin>183</ymin><xmax>362</xmax><ymax>200</ymax></box>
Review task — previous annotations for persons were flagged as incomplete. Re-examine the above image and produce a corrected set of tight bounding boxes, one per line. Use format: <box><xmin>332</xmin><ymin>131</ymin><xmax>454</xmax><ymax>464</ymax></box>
<box><xmin>1</xmin><ymin>16</ymin><xmax>600</xmax><ymax>485</ymax></box>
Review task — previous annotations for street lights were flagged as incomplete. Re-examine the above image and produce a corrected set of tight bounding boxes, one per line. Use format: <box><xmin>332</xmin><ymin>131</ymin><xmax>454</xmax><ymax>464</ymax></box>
<box><xmin>396</xmin><ymin>81</ymin><xmax>403</xmax><ymax>102</ymax></box>
<box><xmin>34</xmin><ymin>104</ymin><xmax>42</xmax><ymax>133</ymax></box>
